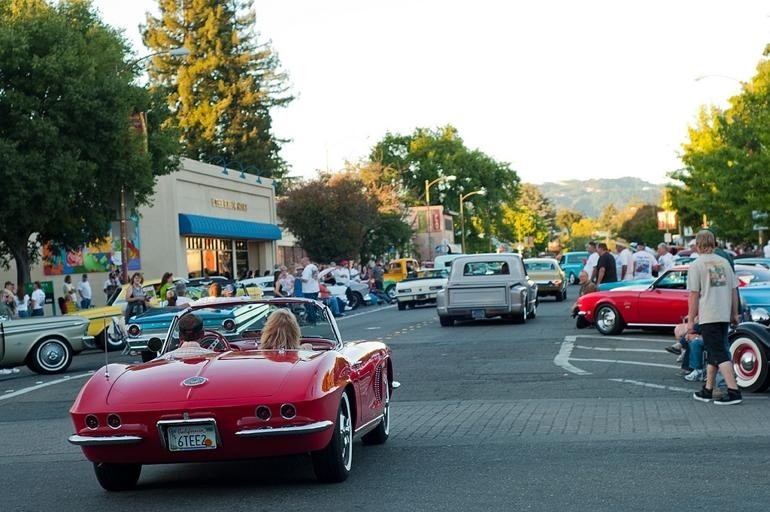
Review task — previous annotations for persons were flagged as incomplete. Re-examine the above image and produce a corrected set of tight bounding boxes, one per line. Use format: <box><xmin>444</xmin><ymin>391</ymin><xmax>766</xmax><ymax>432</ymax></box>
<box><xmin>2</xmin><ymin>281</ymin><xmax>45</xmax><ymax>318</ymax></box>
<box><xmin>77</xmin><ymin>274</ymin><xmax>92</xmax><ymax>309</ymax></box>
<box><xmin>104</xmin><ymin>269</ymin><xmax>122</xmax><ymax>300</ymax></box>
<box><xmin>579</xmin><ymin>228</ymin><xmax>770</xmax><ymax>405</ymax></box>
<box><xmin>238</xmin><ymin>269</ymin><xmax>270</xmax><ymax>281</ymax></box>
<box><xmin>62</xmin><ymin>275</ymin><xmax>77</xmax><ymax>305</ymax></box>
<box><xmin>319</xmin><ymin>283</ymin><xmax>352</xmax><ymax>317</ymax></box>
<box><xmin>274</xmin><ymin>257</ymin><xmax>320</xmax><ymax>326</ymax></box>
<box><xmin>125</xmin><ymin>272</ymin><xmax>148</xmax><ymax>355</ymax></box>
<box><xmin>261</xmin><ymin>308</ymin><xmax>312</xmax><ymax>350</ymax></box>
<box><xmin>210</xmin><ymin>283</ymin><xmax>237</xmax><ymax>297</ymax></box>
<box><xmin>165</xmin><ymin>313</ymin><xmax>214</xmax><ymax>353</ymax></box>
<box><xmin>161</xmin><ymin>272</ymin><xmax>194</xmax><ymax>306</ymax></box>
<box><xmin>314</xmin><ymin>259</ymin><xmax>384</xmax><ymax>290</ymax></box>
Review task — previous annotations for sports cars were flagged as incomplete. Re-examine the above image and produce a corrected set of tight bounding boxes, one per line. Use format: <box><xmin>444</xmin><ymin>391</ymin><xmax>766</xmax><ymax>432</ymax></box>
<box><xmin>65</xmin><ymin>293</ymin><xmax>403</xmax><ymax>497</ymax></box>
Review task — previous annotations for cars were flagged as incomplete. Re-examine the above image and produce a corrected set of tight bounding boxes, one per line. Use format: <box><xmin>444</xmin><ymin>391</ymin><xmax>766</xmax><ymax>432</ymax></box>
<box><xmin>557</xmin><ymin>251</ymin><xmax>592</xmax><ymax>286</ymax></box>
<box><xmin>727</xmin><ymin>308</ymin><xmax>769</xmax><ymax>393</ymax></box>
<box><xmin>435</xmin><ymin>252</ymin><xmax>538</xmax><ymax>328</ymax></box>
<box><xmin>597</xmin><ymin>251</ymin><xmax>770</xmax><ymax>313</ymax></box>
<box><xmin>0</xmin><ymin>313</ymin><xmax>91</xmax><ymax>378</ymax></box>
<box><xmin>55</xmin><ymin>242</ymin><xmax>430</xmax><ymax>336</ymax></box>
<box><xmin>395</xmin><ymin>268</ymin><xmax>451</xmax><ymax>311</ymax></box>
<box><xmin>523</xmin><ymin>257</ymin><xmax>568</xmax><ymax>302</ymax></box>
<box><xmin>571</xmin><ymin>265</ymin><xmax>690</xmax><ymax>336</ymax></box>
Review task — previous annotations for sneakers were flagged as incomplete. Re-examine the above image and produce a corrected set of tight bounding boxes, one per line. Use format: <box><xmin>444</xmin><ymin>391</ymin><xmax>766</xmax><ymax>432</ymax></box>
<box><xmin>692</xmin><ymin>383</ymin><xmax>743</xmax><ymax>405</ymax></box>
<box><xmin>683</xmin><ymin>367</ymin><xmax>708</xmax><ymax>382</ymax></box>
<box><xmin>664</xmin><ymin>346</ymin><xmax>681</xmax><ymax>354</ymax></box>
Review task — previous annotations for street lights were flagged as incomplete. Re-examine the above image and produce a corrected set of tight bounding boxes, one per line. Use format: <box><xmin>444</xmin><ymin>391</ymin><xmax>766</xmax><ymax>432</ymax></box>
<box><xmin>457</xmin><ymin>187</ymin><xmax>489</xmax><ymax>254</ymax></box>
<box><xmin>117</xmin><ymin>46</ymin><xmax>193</xmax><ymax>285</ymax></box>
<box><xmin>424</xmin><ymin>174</ymin><xmax>460</xmax><ymax>260</ymax></box>
